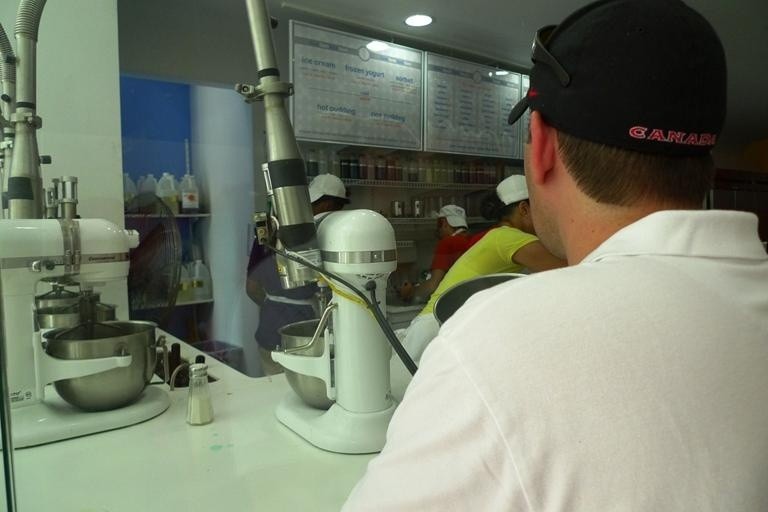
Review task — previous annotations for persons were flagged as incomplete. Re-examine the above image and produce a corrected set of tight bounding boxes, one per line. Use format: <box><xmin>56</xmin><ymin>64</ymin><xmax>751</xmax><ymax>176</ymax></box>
<box><xmin>400</xmin><ymin>204</ymin><xmax>485</xmax><ymax>300</ymax></box>
<box><xmin>416</xmin><ymin>174</ymin><xmax>567</xmax><ymax>321</ymax></box>
<box><xmin>246</xmin><ymin>173</ymin><xmax>352</xmax><ymax>377</ymax></box>
<box><xmin>340</xmin><ymin>0</ymin><xmax>768</xmax><ymax>512</ymax></box>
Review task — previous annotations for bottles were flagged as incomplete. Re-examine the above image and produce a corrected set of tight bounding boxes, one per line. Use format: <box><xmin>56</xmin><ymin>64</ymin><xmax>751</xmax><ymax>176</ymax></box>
<box><xmin>306</xmin><ymin>148</ymin><xmax>496</xmax><ymax>184</ymax></box>
<box><xmin>185</xmin><ymin>364</ymin><xmax>214</xmax><ymax>425</ymax></box>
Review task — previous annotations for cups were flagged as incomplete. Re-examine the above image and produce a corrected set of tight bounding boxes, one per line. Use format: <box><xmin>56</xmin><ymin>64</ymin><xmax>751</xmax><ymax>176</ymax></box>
<box><xmin>390</xmin><ymin>200</ymin><xmax>404</xmax><ymax>218</ymax></box>
<box><xmin>410</xmin><ymin>194</ymin><xmax>474</xmax><ymax>218</ymax></box>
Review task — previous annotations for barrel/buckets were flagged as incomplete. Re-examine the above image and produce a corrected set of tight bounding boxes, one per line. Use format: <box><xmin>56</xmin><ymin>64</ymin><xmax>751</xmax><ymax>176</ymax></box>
<box><xmin>124</xmin><ymin>169</ymin><xmax>199</xmax><ymax>217</ymax></box>
<box><xmin>168</xmin><ymin>259</ymin><xmax>215</xmax><ymax>305</ymax></box>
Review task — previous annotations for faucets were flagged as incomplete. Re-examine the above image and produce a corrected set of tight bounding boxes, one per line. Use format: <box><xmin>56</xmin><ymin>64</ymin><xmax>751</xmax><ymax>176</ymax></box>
<box><xmin>170</xmin><ymin>362</ymin><xmax>189</xmax><ymax>391</ymax></box>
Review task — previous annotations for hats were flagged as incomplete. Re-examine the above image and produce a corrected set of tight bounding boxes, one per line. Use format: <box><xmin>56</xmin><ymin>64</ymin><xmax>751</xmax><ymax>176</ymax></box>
<box><xmin>508</xmin><ymin>0</ymin><xmax>728</xmax><ymax>159</ymax></box>
<box><xmin>436</xmin><ymin>204</ymin><xmax>469</xmax><ymax>230</ymax></box>
<box><xmin>308</xmin><ymin>172</ymin><xmax>351</xmax><ymax>206</ymax></box>
<box><xmin>496</xmin><ymin>174</ymin><xmax>529</xmax><ymax>206</ymax></box>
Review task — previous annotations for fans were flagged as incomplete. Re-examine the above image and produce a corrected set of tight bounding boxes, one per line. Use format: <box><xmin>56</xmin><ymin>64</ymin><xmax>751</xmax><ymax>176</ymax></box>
<box><xmin>122</xmin><ymin>192</ymin><xmax>183</xmax><ymax>336</ymax></box>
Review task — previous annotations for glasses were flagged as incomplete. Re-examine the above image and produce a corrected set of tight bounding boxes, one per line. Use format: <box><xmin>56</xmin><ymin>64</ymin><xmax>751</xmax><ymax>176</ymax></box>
<box><xmin>530</xmin><ymin>24</ymin><xmax>571</xmax><ymax>88</ymax></box>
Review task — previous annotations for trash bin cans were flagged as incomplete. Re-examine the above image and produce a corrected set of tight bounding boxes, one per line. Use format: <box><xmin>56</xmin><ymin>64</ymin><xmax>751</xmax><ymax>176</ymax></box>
<box><xmin>192</xmin><ymin>339</ymin><xmax>242</xmax><ymax>373</ymax></box>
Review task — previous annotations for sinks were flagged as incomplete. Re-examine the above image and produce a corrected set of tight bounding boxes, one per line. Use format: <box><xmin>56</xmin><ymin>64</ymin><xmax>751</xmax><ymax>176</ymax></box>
<box><xmin>152</xmin><ymin>352</ymin><xmax>219</xmax><ymax>389</ymax></box>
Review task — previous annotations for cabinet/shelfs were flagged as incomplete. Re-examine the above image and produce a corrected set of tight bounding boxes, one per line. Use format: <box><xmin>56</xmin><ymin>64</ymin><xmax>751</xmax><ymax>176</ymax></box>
<box><xmin>119</xmin><ymin>210</ymin><xmax>213</xmax><ymax>316</ymax></box>
<box><xmin>302</xmin><ymin>173</ymin><xmax>500</xmax><ymax>256</ymax></box>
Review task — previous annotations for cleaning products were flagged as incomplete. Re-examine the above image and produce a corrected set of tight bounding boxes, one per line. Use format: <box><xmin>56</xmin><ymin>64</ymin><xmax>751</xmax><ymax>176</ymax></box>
<box><xmin>307</xmin><ymin>145</ymin><xmax>506</xmax><ymax>187</ymax></box>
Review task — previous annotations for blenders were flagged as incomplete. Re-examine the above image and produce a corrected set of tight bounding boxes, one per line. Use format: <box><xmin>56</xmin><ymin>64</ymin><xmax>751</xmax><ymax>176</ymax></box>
<box><xmin>234</xmin><ymin>0</ymin><xmax>399</xmax><ymax>455</ymax></box>
<box><xmin>0</xmin><ymin>0</ymin><xmax>170</xmax><ymax>450</ymax></box>
